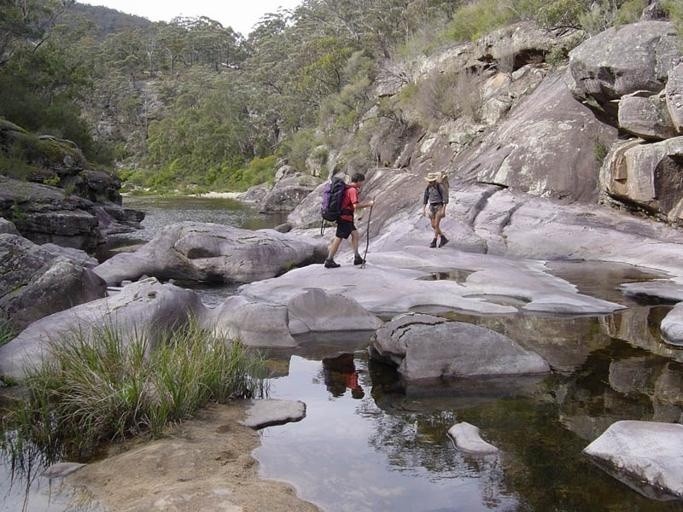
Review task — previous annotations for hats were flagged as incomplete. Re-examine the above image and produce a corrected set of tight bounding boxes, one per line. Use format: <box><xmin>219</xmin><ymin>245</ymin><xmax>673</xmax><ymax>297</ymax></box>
<box><xmin>424</xmin><ymin>173</ymin><xmax>438</xmax><ymax>181</ymax></box>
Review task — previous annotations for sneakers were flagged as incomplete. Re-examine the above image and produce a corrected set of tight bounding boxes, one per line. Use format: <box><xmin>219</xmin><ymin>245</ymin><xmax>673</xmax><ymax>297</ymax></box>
<box><xmin>430</xmin><ymin>237</ymin><xmax>448</xmax><ymax>247</ymax></box>
<box><xmin>325</xmin><ymin>259</ymin><xmax>340</xmax><ymax>267</ymax></box>
<box><xmin>354</xmin><ymin>257</ymin><xmax>365</xmax><ymax>264</ymax></box>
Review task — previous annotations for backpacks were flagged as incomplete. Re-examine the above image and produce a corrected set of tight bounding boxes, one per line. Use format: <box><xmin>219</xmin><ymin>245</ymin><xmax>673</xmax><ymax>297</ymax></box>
<box><xmin>321</xmin><ymin>176</ymin><xmax>353</xmax><ymax>236</ymax></box>
<box><xmin>437</xmin><ymin>170</ymin><xmax>449</xmax><ymax>203</ymax></box>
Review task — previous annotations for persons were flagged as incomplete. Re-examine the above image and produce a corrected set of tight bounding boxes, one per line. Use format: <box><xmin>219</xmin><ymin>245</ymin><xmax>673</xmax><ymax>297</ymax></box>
<box><xmin>323</xmin><ymin>172</ymin><xmax>374</xmax><ymax>268</ymax></box>
<box><xmin>422</xmin><ymin>173</ymin><xmax>450</xmax><ymax>248</ymax></box>
<box><xmin>334</xmin><ymin>354</ymin><xmax>370</xmax><ymax>401</ymax></box>
